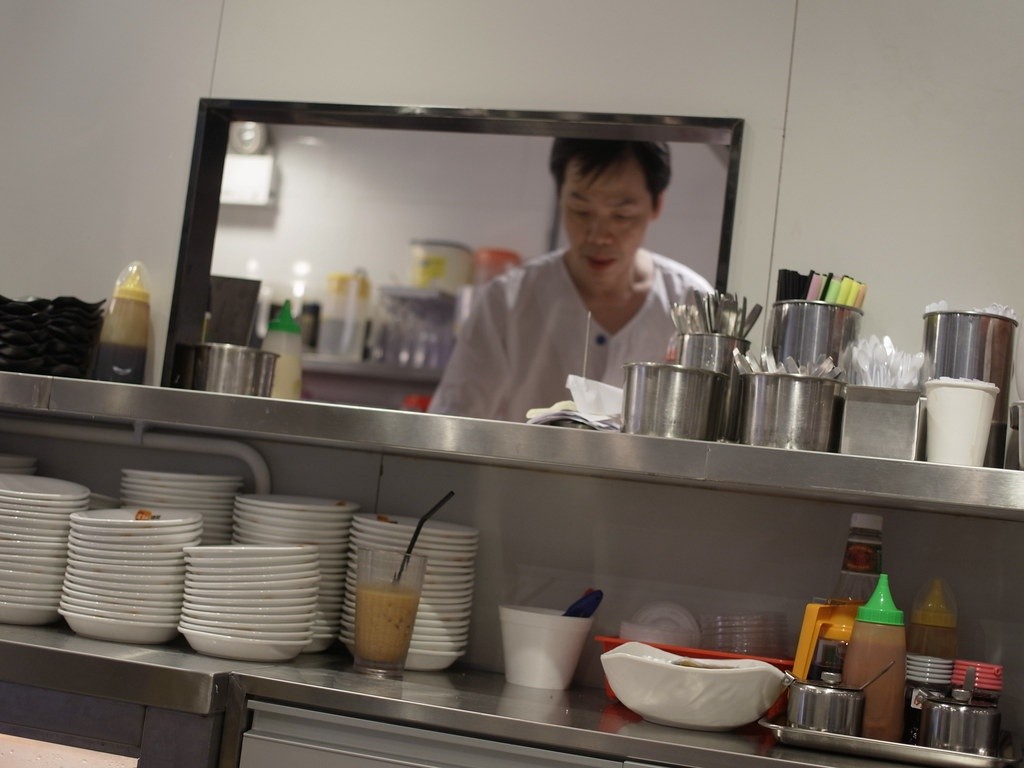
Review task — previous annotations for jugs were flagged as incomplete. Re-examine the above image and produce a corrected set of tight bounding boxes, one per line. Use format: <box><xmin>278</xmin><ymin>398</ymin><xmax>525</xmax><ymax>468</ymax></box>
<box><xmin>318</xmin><ymin>269</ymin><xmax>385</xmax><ymax>363</ymax></box>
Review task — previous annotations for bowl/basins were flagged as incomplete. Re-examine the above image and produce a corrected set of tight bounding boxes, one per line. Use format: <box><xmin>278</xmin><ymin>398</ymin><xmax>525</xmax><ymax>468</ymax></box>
<box><xmin>701</xmin><ymin>612</ymin><xmax>789</xmax><ymax>659</ymax></box>
<box><xmin>601</xmin><ymin>640</ymin><xmax>788</xmax><ymax>732</ymax></box>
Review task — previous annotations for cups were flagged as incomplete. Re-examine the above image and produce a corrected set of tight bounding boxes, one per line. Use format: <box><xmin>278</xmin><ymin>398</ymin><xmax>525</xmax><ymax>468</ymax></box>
<box><xmin>915</xmin><ymin>310</ymin><xmax>1019</xmax><ymax>468</ymax></box>
<box><xmin>622</xmin><ymin>363</ymin><xmax>719</xmax><ymax>442</ymax></box>
<box><xmin>172</xmin><ymin>341</ymin><xmax>280</xmax><ymax>398</ymax></box>
<box><xmin>498</xmin><ymin>604</ymin><xmax>594</xmax><ymax>690</ymax></box>
<box><xmin>738</xmin><ymin>374</ymin><xmax>846</xmax><ymax>452</ymax></box>
<box><xmin>925</xmin><ymin>380</ymin><xmax>1000</xmax><ymax>467</ymax></box>
<box><xmin>771</xmin><ymin>300</ymin><xmax>864</xmax><ymax>384</ymax></box>
<box><xmin>411</xmin><ymin>239</ymin><xmax>475</xmax><ymax>295</ymax></box>
<box><xmin>1015</xmin><ymin>401</ymin><xmax>1024</xmax><ymax>469</ymax></box>
<box><xmin>353</xmin><ymin>547</ymin><xmax>428</xmax><ymax>678</ymax></box>
<box><xmin>476</xmin><ymin>249</ymin><xmax>521</xmax><ymax>289</ymax></box>
<box><xmin>678</xmin><ymin>332</ymin><xmax>751</xmax><ymax>441</ymax></box>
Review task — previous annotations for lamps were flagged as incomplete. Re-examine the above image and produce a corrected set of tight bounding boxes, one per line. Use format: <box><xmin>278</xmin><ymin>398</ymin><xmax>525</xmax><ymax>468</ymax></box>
<box><xmin>228</xmin><ymin>119</ymin><xmax>269</xmax><ymax>156</ymax></box>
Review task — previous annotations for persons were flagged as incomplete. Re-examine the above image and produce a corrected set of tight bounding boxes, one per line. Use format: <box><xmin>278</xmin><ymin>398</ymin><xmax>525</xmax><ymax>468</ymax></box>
<box><xmin>424</xmin><ymin>136</ymin><xmax>715</xmax><ymax>432</ymax></box>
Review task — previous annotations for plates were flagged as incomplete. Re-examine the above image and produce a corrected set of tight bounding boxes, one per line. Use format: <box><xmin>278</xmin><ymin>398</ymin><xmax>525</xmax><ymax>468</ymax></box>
<box><xmin>0</xmin><ymin>454</ymin><xmax>479</xmax><ymax>670</ymax></box>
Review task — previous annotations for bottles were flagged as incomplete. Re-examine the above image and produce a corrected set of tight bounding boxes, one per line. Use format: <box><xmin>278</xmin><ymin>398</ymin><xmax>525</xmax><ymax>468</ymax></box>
<box><xmin>92</xmin><ymin>260</ymin><xmax>150</xmax><ymax>385</ymax></box>
<box><xmin>260</xmin><ymin>300</ymin><xmax>303</xmax><ymax>400</ymax></box>
<box><xmin>841</xmin><ymin>574</ymin><xmax>908</xmax><ymax>742</ymax></box>
<box><xmin>807</xmin><ymin>512</ymin><xmax>885</xmax><ymax>680</ymax></box>
<box><xmin>908</xmin><ymin>575</ymin><xmax>960</xmax><ymax>659</ymax></box>
